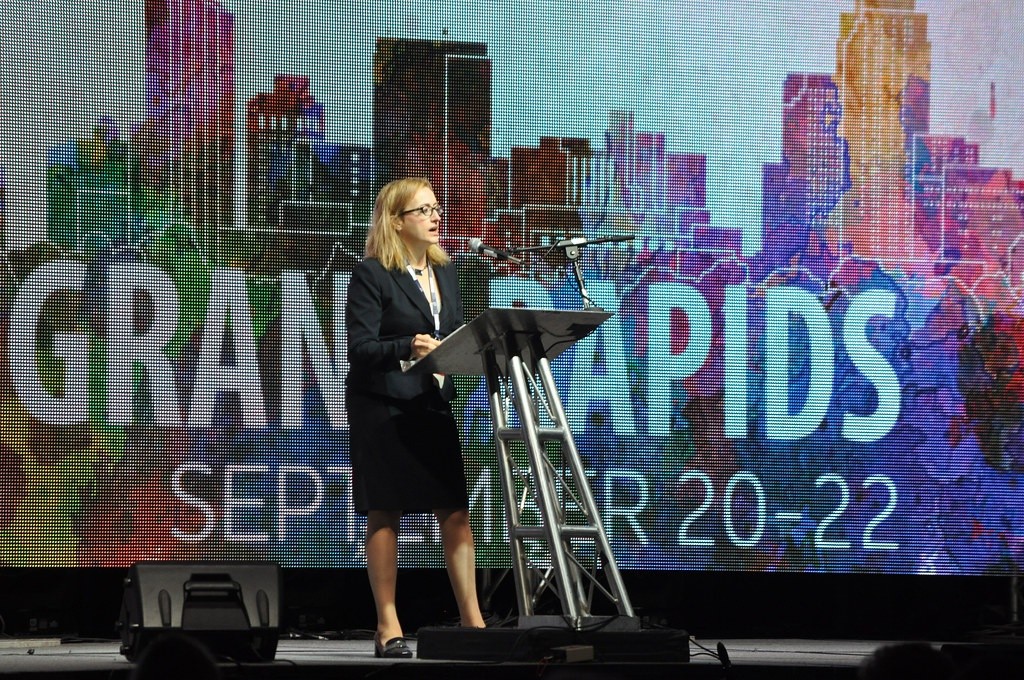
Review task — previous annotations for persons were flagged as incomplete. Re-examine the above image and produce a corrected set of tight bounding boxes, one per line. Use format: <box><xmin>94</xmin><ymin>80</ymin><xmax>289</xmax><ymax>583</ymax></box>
<box><xmin>345</xmin><ymin>178</ymin><xmax>488</xmax><ymax>658</ymax></box>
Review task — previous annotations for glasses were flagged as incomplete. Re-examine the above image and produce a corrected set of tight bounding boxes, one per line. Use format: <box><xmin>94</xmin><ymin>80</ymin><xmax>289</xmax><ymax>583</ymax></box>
<box><xmin>398</xmin><ymin>204</ymin><xmax>444</xmax><ymax>216</ymax></box>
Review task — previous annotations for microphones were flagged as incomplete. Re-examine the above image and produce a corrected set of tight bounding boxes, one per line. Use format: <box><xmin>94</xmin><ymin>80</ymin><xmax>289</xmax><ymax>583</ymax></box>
<box><xmin>468</xmin><ymin>238</ymin><xmax>525</xmax><ymax>265</ymax></box>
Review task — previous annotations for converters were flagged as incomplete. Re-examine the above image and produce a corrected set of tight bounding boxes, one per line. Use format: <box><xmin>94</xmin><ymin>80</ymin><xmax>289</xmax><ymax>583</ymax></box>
<box><xmin>550</xmin><ymin>645</ymin><xmax>593</xmax><ymax>663</ymax></box>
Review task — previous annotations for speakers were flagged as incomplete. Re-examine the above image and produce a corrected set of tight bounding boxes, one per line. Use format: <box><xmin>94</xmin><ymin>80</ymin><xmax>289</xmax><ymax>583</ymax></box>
<box><xmin>115</xmin><ymin>561</ymin><xmax>282</xmax><ymax>662</ymax></box>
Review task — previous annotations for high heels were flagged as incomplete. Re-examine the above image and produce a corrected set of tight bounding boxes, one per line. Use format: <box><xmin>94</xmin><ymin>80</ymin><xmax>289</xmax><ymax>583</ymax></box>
<box><xmin>374</xmin><ymin>629</ymin><xmax>412</xmax><ymax>657</ymax></box>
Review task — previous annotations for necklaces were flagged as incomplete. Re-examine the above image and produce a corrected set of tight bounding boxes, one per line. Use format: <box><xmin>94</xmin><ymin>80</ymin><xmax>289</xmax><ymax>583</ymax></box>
<box><xmin>412</xmin><ymin>265</ymin><xmax>428</xmax><ymax>276</ymax></box>
